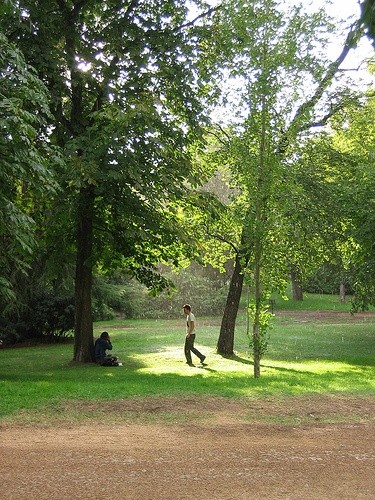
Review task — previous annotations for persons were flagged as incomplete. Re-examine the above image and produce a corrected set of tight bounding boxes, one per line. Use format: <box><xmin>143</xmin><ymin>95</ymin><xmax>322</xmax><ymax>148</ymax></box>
<box><xmin>183</xmin><ymin>304</ymin><xmax>206</xmax><ymax>365</ymax></box>
<box><xmin>93</xmin><ymin>332</ymin><xmax>119</xmax><ymax>366</ymax></box>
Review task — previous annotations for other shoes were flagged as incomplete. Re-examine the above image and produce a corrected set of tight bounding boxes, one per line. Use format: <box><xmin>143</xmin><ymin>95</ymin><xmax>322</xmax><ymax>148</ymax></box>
<box><xmin>186</xmin><ymin>360</ymin><xmax>192</xmax><ymax>364</ymax></box>
<box><xmin>200</xmin><ymin>355</ymin><xmax>206</xmax><ymax>363</ymax></box>
